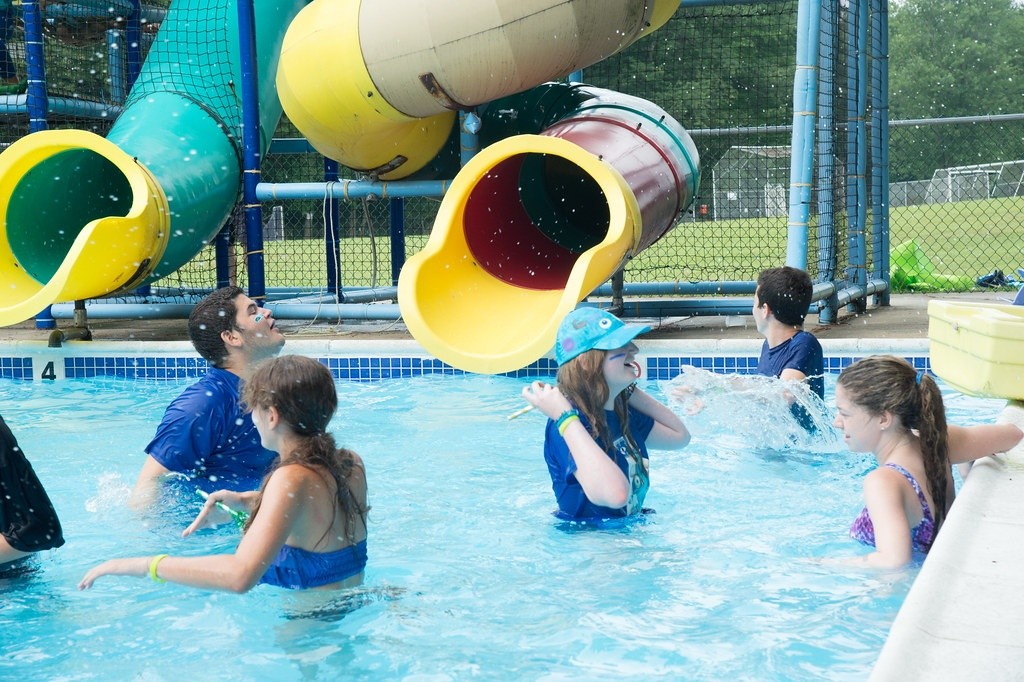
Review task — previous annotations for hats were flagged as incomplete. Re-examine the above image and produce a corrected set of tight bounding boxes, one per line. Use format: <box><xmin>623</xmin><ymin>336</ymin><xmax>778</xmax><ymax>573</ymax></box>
<box><xmin>554</xmin><ymin>306</ymin><xmax>651</xmax><ymax>366</ymax></box>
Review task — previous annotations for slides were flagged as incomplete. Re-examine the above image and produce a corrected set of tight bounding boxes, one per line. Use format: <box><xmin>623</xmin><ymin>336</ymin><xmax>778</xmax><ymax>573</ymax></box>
<box><xmin>0</xmin><ymin>0</ymin><xmax>704</xmax><ymax>378</ymax></box>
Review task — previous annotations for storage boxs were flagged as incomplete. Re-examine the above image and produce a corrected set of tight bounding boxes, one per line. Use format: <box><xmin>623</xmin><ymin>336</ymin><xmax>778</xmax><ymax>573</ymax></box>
<box><xmin>926</xmin><ymin>299</ymin><xmax>1024</xmax><ymax>400</ymax></box>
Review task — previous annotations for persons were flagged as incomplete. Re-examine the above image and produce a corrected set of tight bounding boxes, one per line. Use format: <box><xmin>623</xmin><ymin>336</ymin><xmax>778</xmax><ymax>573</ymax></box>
<box><xmin>673</xmin><ymin>266</ymin><xmax>825</xmax><ymax>441</ymax></box>
<box><xmin>0</xmin><ymin>416</ymin><xmax>66</xmax><ymax>565</ymax></box>
<box><xmin>522</xmin><ymin>307</ymin><xmax>692</xmax><ymax>518</ymax></box>
<box><xmin>832</xmin><ymin>354</ymin><xmax>1024</xmax><ymax>568</ymax></box>
<box><xmin>77</xmin><ymin>354</ymin><xmax>367</xmax><ymax>594</ymax></box>
<box><xmin>140</xmin><ymin>286</ymin><xmax>286</xmax><ymax>477</ymax></box>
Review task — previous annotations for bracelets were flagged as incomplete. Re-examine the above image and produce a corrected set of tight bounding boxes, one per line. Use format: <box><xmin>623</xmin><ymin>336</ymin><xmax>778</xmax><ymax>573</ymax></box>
<box><xmin>149</xmin><ymin>554</ymin><xmax>169</xmax><ymax>584</ymax></box>
<box><xmin>625</xmin><ymin>382</ymin><xmax>638</xmax><ymax>400</ymax></box>
<box><xmin>554</xmin><ymin>408</ymin><xmax>580</xmax><ymax>437</ymax></box>
